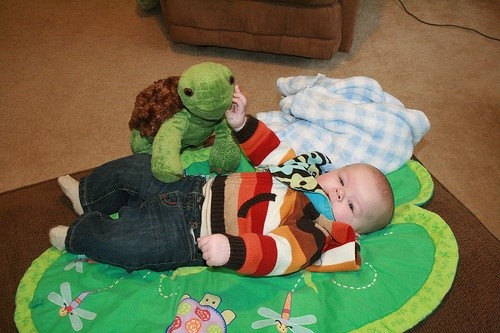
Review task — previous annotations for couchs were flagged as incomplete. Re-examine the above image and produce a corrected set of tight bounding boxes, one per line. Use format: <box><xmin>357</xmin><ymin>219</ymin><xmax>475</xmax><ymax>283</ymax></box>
<box><xmin>157</xmin><ymin>0</ymin><xmax>356</xmax><ymax>60</ymax></box>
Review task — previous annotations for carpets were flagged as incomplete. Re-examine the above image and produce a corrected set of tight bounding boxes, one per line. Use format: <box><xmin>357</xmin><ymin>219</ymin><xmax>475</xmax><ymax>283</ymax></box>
<box><xmin>0</xmin><ymin>134</ymin><xmax>500</xmax><ymax>333</ymax></box>
